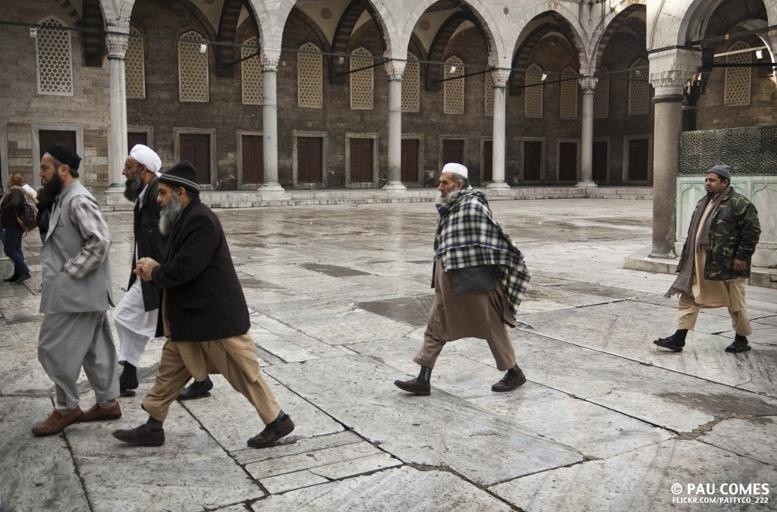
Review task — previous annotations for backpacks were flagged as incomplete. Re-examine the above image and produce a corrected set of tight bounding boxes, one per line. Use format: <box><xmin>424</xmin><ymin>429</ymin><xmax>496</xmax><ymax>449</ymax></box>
<box><xmin>12</xmin><ymin>186</ymin><xmax>38</xmax><ymax>232</ymax></box>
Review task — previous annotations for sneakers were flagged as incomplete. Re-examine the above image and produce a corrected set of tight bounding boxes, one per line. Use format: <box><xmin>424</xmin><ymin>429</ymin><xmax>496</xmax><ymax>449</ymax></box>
<box><xmin>80</xmin><ymin>401</ymin><xmax>121</xmax><ymax>420</ymax></box>
<box><xmin>113</xmin><ymin>425</ymin><xmax>164</xmax><ymax>446</ymax></box>
<box><xmin>31</xmin><ymin>405</ymin><xmax>82</xmax><ymax>435</ymax></box>
<box><xmin>493</xmin><ymin>369</ymin><xmax>526</xmax><ymax>392</ymax></box>
<box><xmin>248</xmin><ymin>413</ymin><xmax>294</xmax><ymax>446</ymax></box>
<box><xmin>3</xmin><ymin>273</ymin><xmax>31</xmax><ymax>282</ymax></box>
<box><xmin>395</xmin><ymin>378</ymin><xmax>430</xmax><ymax>396</ymax></box>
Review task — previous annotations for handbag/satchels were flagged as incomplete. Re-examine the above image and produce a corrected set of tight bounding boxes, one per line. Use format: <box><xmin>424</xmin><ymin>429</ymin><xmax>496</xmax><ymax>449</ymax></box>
<box><xmin>447</xmin><ymin>263</ymin><xmax>494</xmax><ymax>296</ymax></box>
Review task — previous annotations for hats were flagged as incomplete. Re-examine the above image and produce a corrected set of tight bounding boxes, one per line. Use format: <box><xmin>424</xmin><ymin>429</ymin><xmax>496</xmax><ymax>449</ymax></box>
<box><xmin>159</xmin><ymin>160</ymin><xmax>201</xmax><ymax>193</ymax></box>
<box><xmin>48</xmin><ymin>142</ymin><xmax>81</xmax><ymax>170</ymax></box>
<box><xmin>130</xmin><ymin>144</ymin><xmax>161</xmax><ymax>173</ymax></box>
<box><xmin>706</xmin><ymin>164</ymin><xmax>731</xmax><ymax>180</ymax></box>
<box><xmin>442</xmin><ymin>163</ymin><xmax>469</xmax><ymax>179</ymax></box>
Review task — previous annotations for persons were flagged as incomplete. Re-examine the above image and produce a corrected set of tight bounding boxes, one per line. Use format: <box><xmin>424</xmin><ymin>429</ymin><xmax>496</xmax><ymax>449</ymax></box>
<box><xmin>0</xmin><ymin>173</ymin><xmax>35</xmax><ymax>283</ymax></box>
<box><xmin>394</xmin><ymin>162</ymin><xmax>530</xmax><ymax>395</ymax></box>
<box><xmin>112</xmin><ymin>144</ymin><xmax>213</xmax><ymax>401</ymax></box>
<box><xmin>653</xmin><ymin>165</ymin><xmax>761</xmax><ymax>353</ymax></box>
<box><xmin>113</xmin><ymin>160</ymin><xmax>294</xmax><ymax>449</ymax></box>
<box><xmin>31</xmin><ymin>143</ymin><xmax>122</xmax><ymax>433</ymax></box>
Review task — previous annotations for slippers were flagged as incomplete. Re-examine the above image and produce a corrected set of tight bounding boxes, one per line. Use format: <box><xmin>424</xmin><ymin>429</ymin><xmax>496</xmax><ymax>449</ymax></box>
<box><xmin>654</xmin><ymin>338</ymin><xmax>685</xmax><ymax>352</ymax></box>
<box><xmin>180</xmin><ymin>382</ymin><xmax>212</xmax><ymax>400</ymax></box>
<box><xmin>120</xmin><ymin>379</ymin><xmax>138</xmax><ymax>398</ymax></box>
<box><xmin>724</xmin><ymin>339</ymin><xmax>751</xmax><ymax>352</ymax></box>
